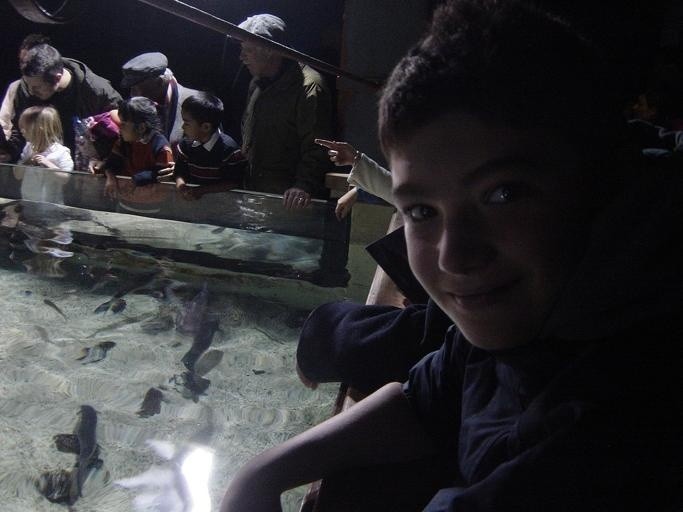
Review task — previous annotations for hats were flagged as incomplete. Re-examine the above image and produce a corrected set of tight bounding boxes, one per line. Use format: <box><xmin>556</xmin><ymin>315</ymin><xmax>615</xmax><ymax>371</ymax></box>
<box><xmin>119</xmin><ymin>52</ymin><xmax>169</xmax><ymax>89</ymax></box>
<box><xmin>226</xmin><ymin>15</ymin><xmax>290</xmax><ymax>43</ymax></box>
